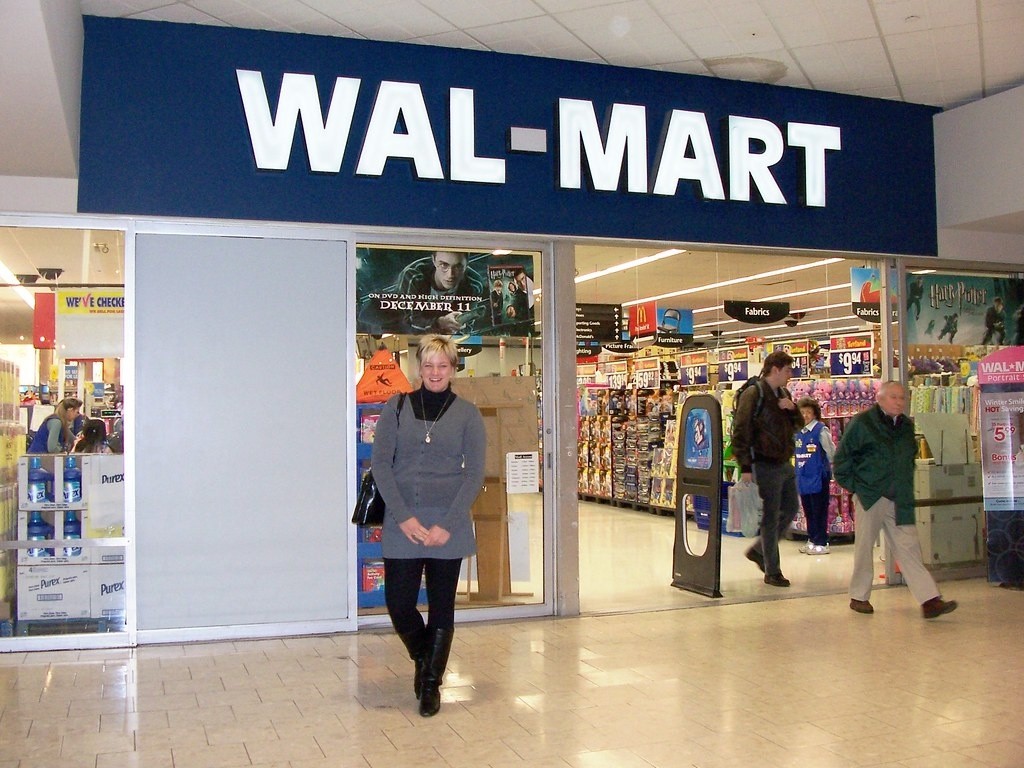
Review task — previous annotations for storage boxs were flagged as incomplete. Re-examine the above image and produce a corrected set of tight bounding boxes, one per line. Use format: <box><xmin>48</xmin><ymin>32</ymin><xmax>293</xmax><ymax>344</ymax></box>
<box><xmin>15</xmin><ymin>451</ymin><xmax>129</xmax><ymax>622</ymax></box>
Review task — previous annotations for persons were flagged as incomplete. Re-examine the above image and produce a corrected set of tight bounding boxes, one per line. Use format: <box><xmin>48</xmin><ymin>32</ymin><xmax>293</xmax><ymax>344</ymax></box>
<box><xmin>981</xmin><ymin>297</ymin><xmax>1005</xmax><ymax>345</ymax></box>
<box><xmin>71</xmin><ymin>419</ymin><xmax>114</xmax><ymax>454</ymax></box>
<box><xmin>356</xmin><ymin>252</ymin><xmax>485</xmax><ymax>333</ymax></box>
<box><xmin>937</xmin><ymin>313</ymin><xmax>958</xmax><ymax>345</ymax></box>
<box><xmin>833</xmin><ymin>379</ymin><xmax>958</xmax><ymax>618</ymax></box>
<box><xmin>730</xmin><ymin>350</ymin><xmax>805</xmax><ymax>587</ymax></box>
<box><xmin>27</xmin><ymin>398</ymin><xmax>89</xmax><ymax>454</ymax></box>
<box><xmin>371</xmin><ymin>333</ymin><xmax>487</xmax><ymax>717</ymax></box>
<box><xmin>907</xmin><ymin>277</ymin><xmax>924</xmax><ymax>320</ymax></box>
<box><xmin>794</xmin><ymin>398</ymin><xmax>835</xmax><ymax>555</ymax></box>
<box><xmin>491</xmin><ymin>268</ymin><xmax>529</xmax><ymax>325</ymax></box>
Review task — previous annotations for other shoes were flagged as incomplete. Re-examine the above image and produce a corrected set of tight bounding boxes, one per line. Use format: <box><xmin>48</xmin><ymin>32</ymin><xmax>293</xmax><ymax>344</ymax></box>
<box><xmin>799</xmin><ymin>539</ymin><xmax>814</xmax><ymax>553</ymax></box>
<box><xmin>745</xmin><ymin>547</ymin><xmax>765</xmax><ymax>573</ymax></box>
<box><xmin>806</xmin><ymin>543</ymin><xmax>830</xmax><ymax>555</ymax></box>
<box><xmin>765</xmin><ymin>573</ymin><xmax>790</xmax><ymax>587</ymax></box>
<box><xmin>850</xmin><ymin>598</ymin><xmax>873</xmax><ymax>613</ymax></box>
<box><xmin>924</xmin><ymin>598</ymin><xmax>956</xmax><ymax>618</ymax></box>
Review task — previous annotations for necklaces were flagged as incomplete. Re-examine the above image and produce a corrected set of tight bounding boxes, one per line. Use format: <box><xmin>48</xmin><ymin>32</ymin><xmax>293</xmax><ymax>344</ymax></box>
<box><xmin>421</xmin><ymin>391</ymin><xmax>451</xmax><ymax>443</ymax></box>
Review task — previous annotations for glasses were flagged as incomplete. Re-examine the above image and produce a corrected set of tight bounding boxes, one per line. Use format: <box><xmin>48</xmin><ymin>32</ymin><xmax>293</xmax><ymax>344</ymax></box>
<box><xmin>434</xmin><ymin>263</ymin><xmax>467</xmax><ymax>272</ymax></box>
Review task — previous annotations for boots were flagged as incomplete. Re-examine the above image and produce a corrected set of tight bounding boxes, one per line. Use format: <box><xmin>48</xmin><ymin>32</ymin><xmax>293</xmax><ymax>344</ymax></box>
<box><xmin>395</xmin><ymin>615</ymin><xmax>426</xmax><ymax>700</ymax></box>
<box><xmin>419</xmin><ymin>624</ymin><xmax>454</xmax><ymax>717</ymax></box>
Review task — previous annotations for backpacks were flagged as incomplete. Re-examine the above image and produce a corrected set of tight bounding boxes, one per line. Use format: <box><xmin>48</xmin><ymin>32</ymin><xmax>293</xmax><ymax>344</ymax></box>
<box><xmin>731</xmin><ymin>376</ymin><xmax>794</xmax><ymax>427</ymax></box>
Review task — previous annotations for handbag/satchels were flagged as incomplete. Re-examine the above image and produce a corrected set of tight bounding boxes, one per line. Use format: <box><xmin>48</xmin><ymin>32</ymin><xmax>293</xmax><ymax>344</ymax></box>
<box><xmin>726</xmin><ymin>478</ymin><xmax>763</xmax><ymax>538</ymax></box>
<box><xmin>351</xmin><ymin>392</ymin><xmax>407</xmax><ymax>528</ymax></box>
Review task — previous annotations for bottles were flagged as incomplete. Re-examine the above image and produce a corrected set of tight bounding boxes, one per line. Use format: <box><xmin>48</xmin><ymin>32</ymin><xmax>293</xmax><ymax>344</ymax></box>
<box><xmin>28</xmin><ymin>457</ymin><xmax>54</xmax><ymax>504</ymax></box>
<box><xmin>64</xmin><ymin>509</ymin><xmax>80</xmax><ymax>555</ymax></box>
<box><xmin>28</xmin><ymin>511</ymin><xmax>55</xmax><ymax>557</ymax></box>
<box><xmin>64</xmin><ymin>457</ymin><xmax>82</xmax><ymax>502</ymax></box>
